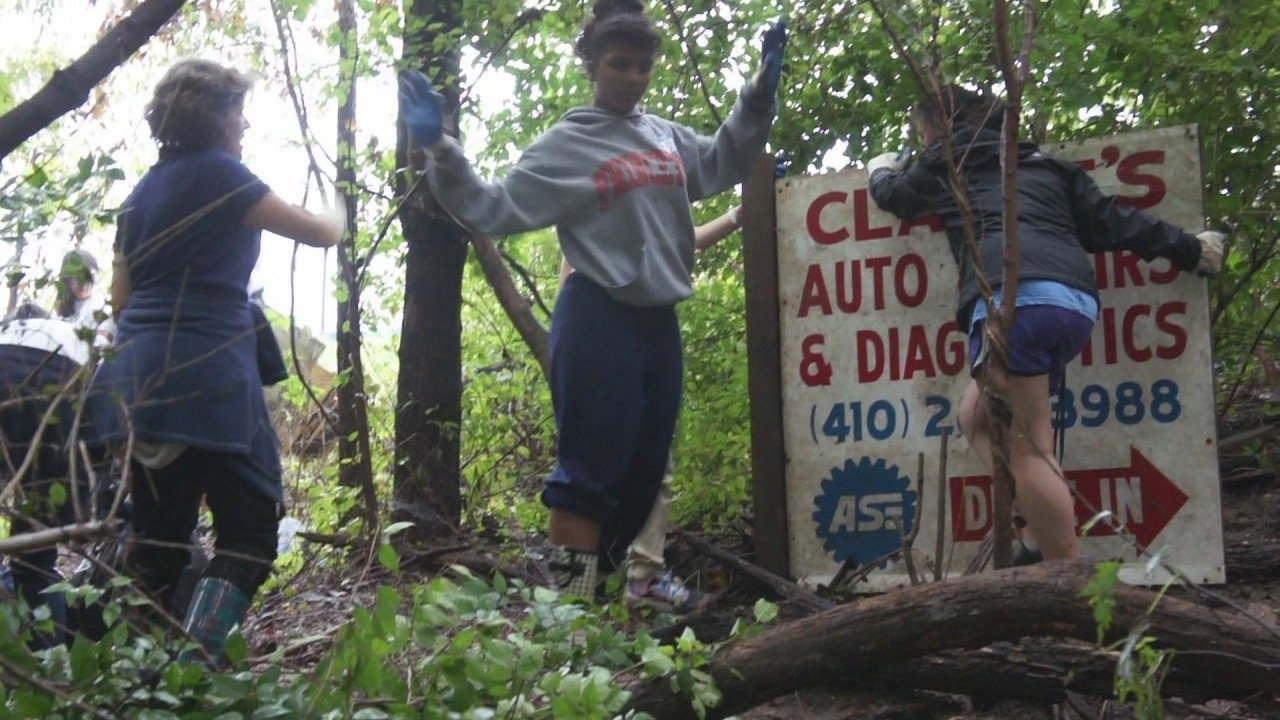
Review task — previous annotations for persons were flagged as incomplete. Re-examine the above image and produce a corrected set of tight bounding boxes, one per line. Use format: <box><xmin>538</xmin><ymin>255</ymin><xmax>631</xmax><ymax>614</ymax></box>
<box><xmin>247</xmin><ymin>301</ymin><xmax>341</xmax><ymax>474</ymax></box>
<box><xmin>0</xmin><ymin>302</ymin><xmax>78</xmax><ymax>649</ymax></box>
<box><xmin>79</xmin><ymin>60</ymin><xmax>348</xmax><ymax>674</ymax></box>
<box><xmin>394</xmin><ymin>0</ymin><xmax>786</xmax><ymax>672</ymax></box>
<box><xmin>869</xmin><ymin>86</ymin><xmax>1222</xmax><ymax>560</ymax></box>
<box><xmin>48</xmin><ymin>250</ymin><xmax>131</xmax><ymax>545</ymax></box>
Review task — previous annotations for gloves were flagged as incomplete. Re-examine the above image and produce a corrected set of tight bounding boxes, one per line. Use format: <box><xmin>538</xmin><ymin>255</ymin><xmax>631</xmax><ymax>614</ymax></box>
<box><xmin>397</xmin><ymin>68</ymin><xmax>445</xmax><ymax>148</ymax></box>
<box><xmin>750</xmin><ymin>17</ymin><xmax>788</xmax><ymax>93</ymax></box>
<box><xmin>1197</xmin><ymin>232</ymin><xmax>1224</xmax><ymax>276</ymax></box>
<box><xmin>867</xmin><ymin>147</ymin><xmax>912</xmax><ymax>173</ymax></box>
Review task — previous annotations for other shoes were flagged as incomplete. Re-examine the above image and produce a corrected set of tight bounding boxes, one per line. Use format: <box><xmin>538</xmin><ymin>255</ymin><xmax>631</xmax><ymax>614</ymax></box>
<box><xmin>622</xmin><ymin>571</ymin><xmax>709</xmax><ymax>618</ymax></box>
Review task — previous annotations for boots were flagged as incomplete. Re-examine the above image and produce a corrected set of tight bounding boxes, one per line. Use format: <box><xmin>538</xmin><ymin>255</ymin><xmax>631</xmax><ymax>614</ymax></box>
<box><xmin>180</xmin><ymin>575</ymin><xmax>249</xmax><ymax>669</ymax></box>
